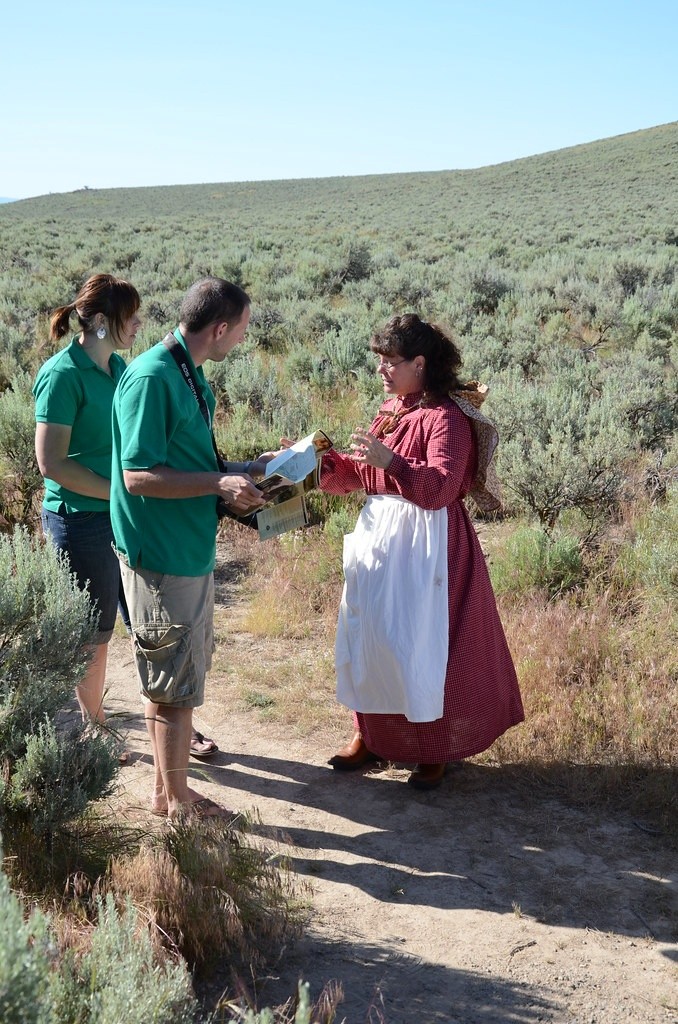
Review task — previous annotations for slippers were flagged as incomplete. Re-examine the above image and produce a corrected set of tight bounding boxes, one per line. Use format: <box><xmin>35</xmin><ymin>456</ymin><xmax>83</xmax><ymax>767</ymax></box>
<box><xmin>151</xmin><ymin>798</ymin><xmax>241</xmax><ymax>822</ymax></box>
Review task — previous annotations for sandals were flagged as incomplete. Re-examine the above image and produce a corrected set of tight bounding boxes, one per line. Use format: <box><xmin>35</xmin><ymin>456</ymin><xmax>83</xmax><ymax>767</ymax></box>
<box><xmin>189</xmin><ymin>731</ymin><xmax>219</xmax><ymax>757</ymax></box>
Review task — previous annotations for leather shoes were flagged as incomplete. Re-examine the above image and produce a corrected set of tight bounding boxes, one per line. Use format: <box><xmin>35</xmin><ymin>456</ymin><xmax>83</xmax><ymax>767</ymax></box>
<box><xmin>326</xmin><ymin>730</ymin><xmax>382</xmax><ymax>768</ymax></box>
<box><xmin>408</xmin><ymin>761</ymin><xmax>448</xmax><ymax>789</ymax></box>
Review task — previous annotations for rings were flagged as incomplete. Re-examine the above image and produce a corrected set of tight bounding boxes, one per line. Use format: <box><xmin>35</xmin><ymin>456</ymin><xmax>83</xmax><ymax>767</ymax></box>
<box><xmin>362</xmin><ymin>448</ymin><xmax>370</xmax><ymax>455</ymax></box>
<box><xmin>371</xmin><ymin>441</ymin><xmax>380</xmax><ymax>447</ymax></box>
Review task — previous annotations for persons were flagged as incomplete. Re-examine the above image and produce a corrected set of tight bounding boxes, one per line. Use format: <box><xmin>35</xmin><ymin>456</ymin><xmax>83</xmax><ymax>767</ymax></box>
<box><xmin>109</xmin><ymin>277</ymin><xmax>288</xmax><ymax>821</ymax></box>
<box><xmin>30</xmin><ymin>274</ymin><xmax>141</xmax><ymax>766</ymax></box>
<box><xmin>279</xmin><ymin>312</ymin><xmax>492</xmax><ymax>789</ymax></box>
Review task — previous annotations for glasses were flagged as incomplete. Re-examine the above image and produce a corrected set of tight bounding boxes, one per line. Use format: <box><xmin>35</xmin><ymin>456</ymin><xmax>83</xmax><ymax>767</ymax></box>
<box><xmin>372</xmin><ymin>355</ymin><xmax>408</xmax><ymax>369</ymax></box>
